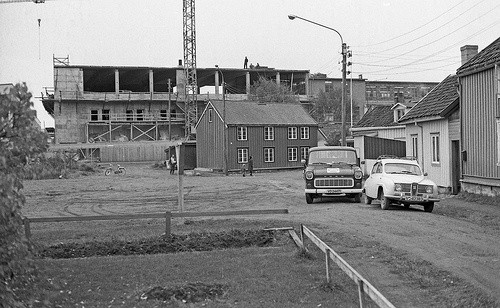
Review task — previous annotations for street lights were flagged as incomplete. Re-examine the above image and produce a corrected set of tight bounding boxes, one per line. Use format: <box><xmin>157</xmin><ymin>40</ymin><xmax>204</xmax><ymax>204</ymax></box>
<box><xmin>214</xmin><ymin>64</ymin><xmax>229</xmax><ymax>176</ymax></box>
<box><xmin>287</xmin><ymin>13</ymin><xmax>353</xmax><ymax>146</ymax></box>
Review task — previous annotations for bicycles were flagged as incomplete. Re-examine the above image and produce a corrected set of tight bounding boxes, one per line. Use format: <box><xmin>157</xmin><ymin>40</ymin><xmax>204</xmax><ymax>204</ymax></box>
<box><xmin>103</xmin><ymin>162</ymin><xmax>126</xmax><ymax>175</ymax></box>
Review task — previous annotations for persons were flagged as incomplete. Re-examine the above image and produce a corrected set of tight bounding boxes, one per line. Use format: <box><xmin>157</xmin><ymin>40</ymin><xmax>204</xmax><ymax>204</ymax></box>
<box><xmin>240</xmin><ymin>163</ymin><xmax>248</xmax><ymax>177</ymax></box>
<box><xmin>243</xmin><ymin>56</ymin><xmax>249</xmax><ymax>70</ymax></box>
<box><xmin>168</xmin><ymin>154</ymin><xmax>177</xmax><ymax>175</ymax></box>
<box><xmin>247</xmin><ymin>156</ymin><xmax>254</xmax><ymax>176</ymax></box>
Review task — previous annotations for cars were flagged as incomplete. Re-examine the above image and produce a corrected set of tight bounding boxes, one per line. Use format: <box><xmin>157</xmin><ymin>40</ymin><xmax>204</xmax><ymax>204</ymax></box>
<box><xmin>362</xmin><ymin>157</ymin><xmax>440</xmax><ymax>213</ymax></box>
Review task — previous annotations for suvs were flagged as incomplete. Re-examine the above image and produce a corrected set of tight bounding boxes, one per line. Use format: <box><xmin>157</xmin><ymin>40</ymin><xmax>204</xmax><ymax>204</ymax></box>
<box><xmin>302</xmin><ymin>146</ymin><xmax>362</xmax><ymax>201</ymax></box>
